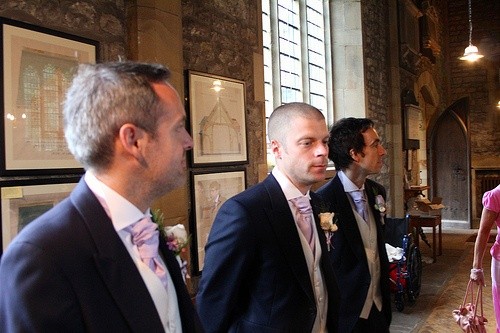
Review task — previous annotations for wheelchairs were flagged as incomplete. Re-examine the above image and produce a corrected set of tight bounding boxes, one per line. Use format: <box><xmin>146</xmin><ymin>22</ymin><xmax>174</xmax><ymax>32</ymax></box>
<box><xmin>382</xmin><ymin>215</ymin><xmax>433</xmax><ymax>313</ymax></box>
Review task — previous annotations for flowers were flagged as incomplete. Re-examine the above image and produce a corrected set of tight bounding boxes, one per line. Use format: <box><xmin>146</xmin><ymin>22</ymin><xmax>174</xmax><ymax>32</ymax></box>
<box><xmin>373</xmin><ymin>189</ymin><xmax>390</xmax><ymax>225</ymax></box>
<box><xmin>318</xmin><ymin>212</ymin><xmax>338</xmax><ymax>250</ymax></box>
<box><xmin>149</xmin><ymin>208</ymin><xmax>193</xmax><ymax>285</ymax></box>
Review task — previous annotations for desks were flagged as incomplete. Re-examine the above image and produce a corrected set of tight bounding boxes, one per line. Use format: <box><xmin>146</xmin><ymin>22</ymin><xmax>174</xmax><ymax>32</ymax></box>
<box><xmin>409</xmin><ymin>214</ymin><xmax>442</xmax><ymax>263</ymax></box>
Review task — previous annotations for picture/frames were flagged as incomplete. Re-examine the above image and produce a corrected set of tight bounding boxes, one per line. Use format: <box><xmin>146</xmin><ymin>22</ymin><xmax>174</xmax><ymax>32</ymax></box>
<box><xmin>184</xmin><ymin>68</ymin><xmax>249</xmax><ymax>168</ymax></box>
<box><xmin>0</xmin><ymin>15</ymin><xmax>100</xmax><ymax>177</ymax></box>
<box><xmin>189</xmin><ymin>166</ymin><xmax>248</xmax><ymax>275</ymax></box>
<box><xmin>0</xmin><ymin>176</ymin><xmax>82</xmax><ymax>261</ymax></box>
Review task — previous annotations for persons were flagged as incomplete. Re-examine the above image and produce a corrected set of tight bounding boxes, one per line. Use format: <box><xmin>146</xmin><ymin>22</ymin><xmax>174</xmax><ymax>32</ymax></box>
<box><xmin>0</xmin><ymin>59</ymin><xmax>205</xmax><ymax>333</ymax></box>
<box><xmin>194</xmin><ymin>102</ymin><xmax>335</xmax><ymax>333</ymax></box>
<box><xmin>317</xmin><ymin>117</ymin><xmax>392</xmax><ymax>333</ymax></box>
<box><xmin>470</xmin><ymin>185</ymin><xmax>500</xmax><ymax>333</ymax></box>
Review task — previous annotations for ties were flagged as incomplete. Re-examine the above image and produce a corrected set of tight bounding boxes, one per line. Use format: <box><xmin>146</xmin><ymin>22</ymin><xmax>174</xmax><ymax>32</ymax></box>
<box><xmin>96</xmin><ymin>196</ymin><xmax>168</xmax><ymax>294</ymax></box>
<box><xmin>290</xmin><ymin>197</ymin><xmax>313</xmax><ymax>244</ymax></box>
<box><xmin>347</xmin><ymin>190</ymin><xmax>370</xmax><ymax>227</ymax></box>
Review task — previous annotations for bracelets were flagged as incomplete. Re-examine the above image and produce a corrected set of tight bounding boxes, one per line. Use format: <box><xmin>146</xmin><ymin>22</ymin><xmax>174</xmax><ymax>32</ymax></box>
<box><xmin>471</xmin><ymin>268</ymin><xmax>484</xmax><ymax>273</ymax></box>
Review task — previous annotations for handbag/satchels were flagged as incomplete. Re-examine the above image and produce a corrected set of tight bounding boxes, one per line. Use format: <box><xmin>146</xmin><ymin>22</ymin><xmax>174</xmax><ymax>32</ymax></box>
<box><xmin>453</xmin><ymin>279</ymin><xmax>488</xmax><ymax>333</ymax></box>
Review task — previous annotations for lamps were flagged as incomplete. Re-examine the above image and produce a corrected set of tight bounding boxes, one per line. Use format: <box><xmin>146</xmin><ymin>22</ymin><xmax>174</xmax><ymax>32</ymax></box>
<box><xmin>456</xmin><ymin>0</ymin><xmax>485</xmax><ymax>61</ymax></box>
